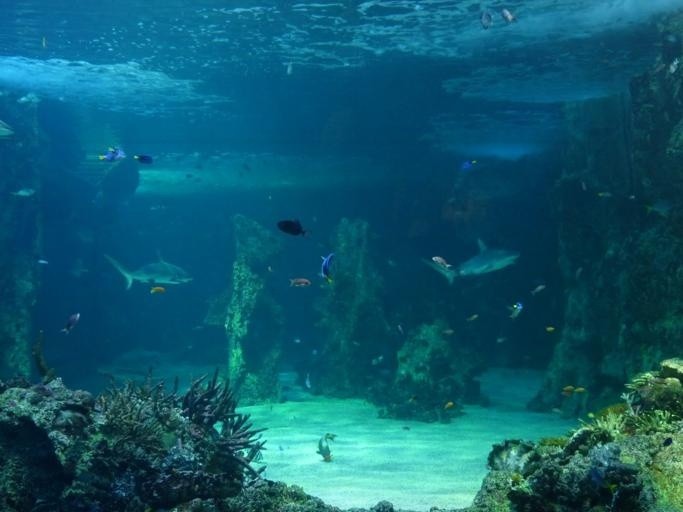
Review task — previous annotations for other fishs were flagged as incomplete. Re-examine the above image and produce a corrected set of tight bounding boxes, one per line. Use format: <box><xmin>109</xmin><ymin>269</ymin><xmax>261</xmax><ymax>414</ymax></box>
<box><xmin>11</xmin><ymin>8</ymin><xmax>635</xmax><ymax>411</ymax></box>
